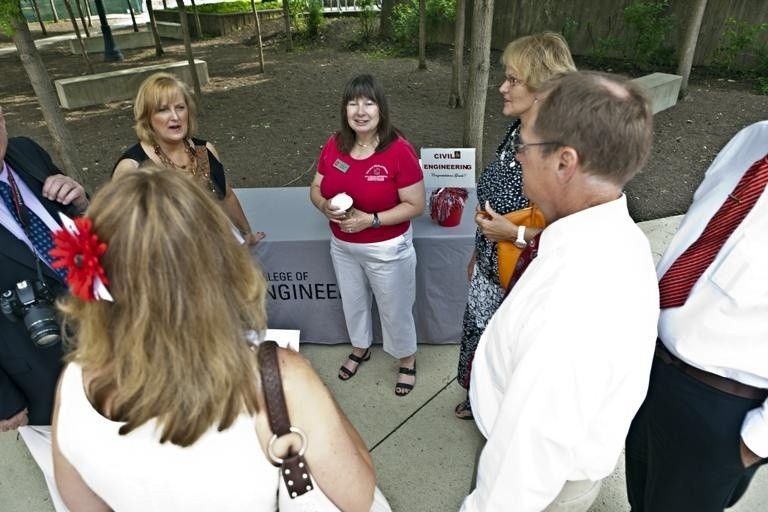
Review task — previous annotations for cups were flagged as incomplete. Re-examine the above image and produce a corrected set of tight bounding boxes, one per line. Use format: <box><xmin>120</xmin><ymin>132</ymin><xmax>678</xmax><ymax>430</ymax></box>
<box><xmin>330</xmin><ymin>193</ymin><xmax>354</xmax><ymax>222</ymax></box>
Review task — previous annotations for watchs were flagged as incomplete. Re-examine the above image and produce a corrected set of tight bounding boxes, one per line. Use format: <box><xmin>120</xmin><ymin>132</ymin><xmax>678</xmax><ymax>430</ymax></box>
<box><xmin>515</xmin><ymin>225</ymin><xmax>528</xmax><ymax>249</ymax></box>
<box><xmin>371</xmin><ymin>212</ymin><xmax>381</xmax><ymax>229</ymax></box>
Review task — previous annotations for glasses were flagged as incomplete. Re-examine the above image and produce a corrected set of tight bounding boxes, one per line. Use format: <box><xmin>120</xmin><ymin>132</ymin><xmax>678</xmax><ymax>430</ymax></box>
<box><xmin>513</xmin><ymin>141</ymin><xmax>564</xmax><ymax>152</ymax></box>
<box><xmin>505</xmin><ymin>75</ymin><xmax>527</xmax><ymax>86</ymax></box>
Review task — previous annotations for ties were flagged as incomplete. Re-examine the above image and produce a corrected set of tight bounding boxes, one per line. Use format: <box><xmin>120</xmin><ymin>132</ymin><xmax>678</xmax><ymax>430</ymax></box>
<box><xmin>658</xmin><ymin>154</ymin><xmax>768</xmax><ymax>312</ymax></box>
<box><xmin>0</xmin><ymin>181</ymin><xmax>70</xmax><ymax>278</ymax></box>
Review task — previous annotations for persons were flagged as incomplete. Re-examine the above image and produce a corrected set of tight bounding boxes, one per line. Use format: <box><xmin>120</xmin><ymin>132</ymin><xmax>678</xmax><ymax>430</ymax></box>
<box><xmin>626</xmin><ymin>121</ymin><xmax>768</xmax><ymax>512</ymax></box>
<box><xmin>309</xmin><ymin>73</ymin><xmax>426</xmax><ymax>396</ymax></box>
<box><xmin>454</xmin><ymin>30</ymin><xmax>576</xmax><ymax>421</ymax></box>
<box><xmin>0</xmin><ymin>108</ymin><xmax>91</xmax><ymax>512</ymax></box>
<box><xmin>456</xmin><ymin>71</ymin><xmax>660</xmax><ymax>512</ymax></box>
<box><xmin>51</xmin><ymin>168</ymin><xmax>395</xmax><ymax>510</ymax></box>
<box><xmin>109</xmin><ymin>71</ymin><xmax>267</xmax><ymax>246</ymax></box>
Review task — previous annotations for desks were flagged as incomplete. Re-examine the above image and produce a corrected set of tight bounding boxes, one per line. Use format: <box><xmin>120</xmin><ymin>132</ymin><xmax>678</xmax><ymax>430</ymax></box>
<box><xmin>214</xmin><ymin>185</ymin><xmax>487</xmax><ymax>346</ymax></box>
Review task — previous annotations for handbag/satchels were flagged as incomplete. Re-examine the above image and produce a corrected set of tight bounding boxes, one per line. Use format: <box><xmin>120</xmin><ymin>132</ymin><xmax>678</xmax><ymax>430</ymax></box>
<box><xmin>256</xmin><ymin>339</ymin><xmax>392</xmax><ymax>512</ymax></box>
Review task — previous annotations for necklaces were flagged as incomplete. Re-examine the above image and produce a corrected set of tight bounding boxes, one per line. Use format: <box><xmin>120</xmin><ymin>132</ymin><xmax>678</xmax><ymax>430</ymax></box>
<box><xmin>149</xmin><ymin>135</ymin><xmax>217</xmax><ymax>199</ymax></box>
<box><xmin>354</xmin><ymin>132</ymin><xmax>380</xmax><ymax>149</ymax></box>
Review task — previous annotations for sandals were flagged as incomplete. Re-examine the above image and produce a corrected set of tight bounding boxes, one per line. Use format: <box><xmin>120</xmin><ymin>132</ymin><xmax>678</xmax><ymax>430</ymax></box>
<box><xmin>395</xmin><ymin>356</ymin><xmax>417</xmax><ymax>397</ymax></box>
<box><xmin>338</xmin><ymin>348</ymin><xmax>371</xmax><ymax>381</ymax></box>
<box><xmin>455</xmin><ymin>401</ymin><xmax>475</xmax><ymax>421</ymax></box>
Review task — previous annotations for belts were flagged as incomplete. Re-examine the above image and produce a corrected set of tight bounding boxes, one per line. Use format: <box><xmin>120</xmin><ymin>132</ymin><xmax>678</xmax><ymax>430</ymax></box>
<box><xmin>655</xmin><ymin>343</ymin><xmax>765</xmax><ymax>400</ymax></box>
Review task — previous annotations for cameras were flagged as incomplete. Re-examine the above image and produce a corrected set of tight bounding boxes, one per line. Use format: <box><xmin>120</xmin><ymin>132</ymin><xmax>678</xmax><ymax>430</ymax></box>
<box><xmin>0</xmin><ymin>278</ymin><xmax>62</xmax><ymax>351</ymax></box>
